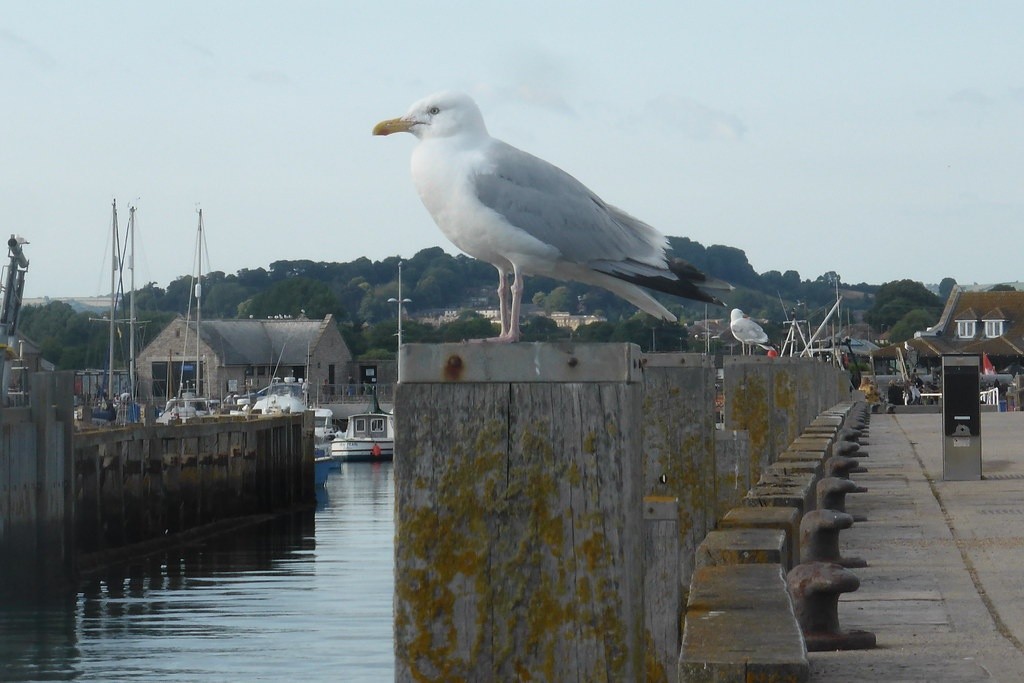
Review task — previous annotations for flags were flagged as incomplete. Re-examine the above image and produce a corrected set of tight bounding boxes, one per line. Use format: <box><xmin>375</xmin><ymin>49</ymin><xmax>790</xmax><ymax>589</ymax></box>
<box><xmin>983</xmin><ymin>351</ymin><xmax>998</xmax><ymax>375</ymax></box>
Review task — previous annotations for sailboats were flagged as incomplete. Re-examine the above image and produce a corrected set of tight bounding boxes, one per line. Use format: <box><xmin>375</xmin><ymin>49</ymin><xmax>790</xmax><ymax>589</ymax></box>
<box><xmin>74</xmin><ymin>194</ymin><xmax>341</xmax><ymax>462</ymax></box>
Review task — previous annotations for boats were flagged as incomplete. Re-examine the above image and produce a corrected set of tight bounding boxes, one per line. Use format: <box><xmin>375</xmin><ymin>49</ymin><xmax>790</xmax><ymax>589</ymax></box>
<box><xmin>328</xmin><ymin>385</ymin><xmax>395</xmax><ymax>462</ymax></box>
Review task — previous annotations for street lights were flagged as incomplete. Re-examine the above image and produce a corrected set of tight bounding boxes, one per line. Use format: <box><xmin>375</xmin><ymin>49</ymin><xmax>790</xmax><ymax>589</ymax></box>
<box><xmin>386</xmin><ymin>260</ymin><xmax>414</xmax><ymax>383</ymax></box>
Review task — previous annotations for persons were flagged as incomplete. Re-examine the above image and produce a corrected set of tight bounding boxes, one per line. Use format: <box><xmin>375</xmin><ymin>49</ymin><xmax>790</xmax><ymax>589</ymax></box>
<box><xmin>113</xmin><ymin>394</ymin><xmax>121</xmax><ymax>409</ymax></box>
<box><xmin>301</xmin><ymin>379</ymin><xmax>309</xmax><ymax>403</ymax></box>
<box><xmin>906</xmin><ymin>369</ymin><xmax>924</xmax><ymax>405</ymax></box>
<box><xmin>980</xmin><ymin>377</ymin><xmax>1000</xmax><ymax>389</ymax></box>
<box><xmin>347</xmin><ymin>374</ymin><xmax>356</xmax><ymax>397</ymax></box>
<box><xmin>889</xmin><ymin>379</ymin><xmax>895</xmax><ymax>386</ymax></box>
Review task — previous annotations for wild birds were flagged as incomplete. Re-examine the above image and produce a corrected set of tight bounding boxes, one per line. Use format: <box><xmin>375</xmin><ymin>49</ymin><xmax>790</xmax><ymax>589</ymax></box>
<box><xmin>728</xmin><ymin>304</ymin><xmax>777</xmax><ymax>356</ymax></box>
<box><xmin>371</xmin><ymin>90</ymin><xmax>735</xmax><ymax>343</ymax></box>
<box><xmin>16</xmin><ymin>234</ymin><xmax>30</xmax><ymax>246</ymax></box>
